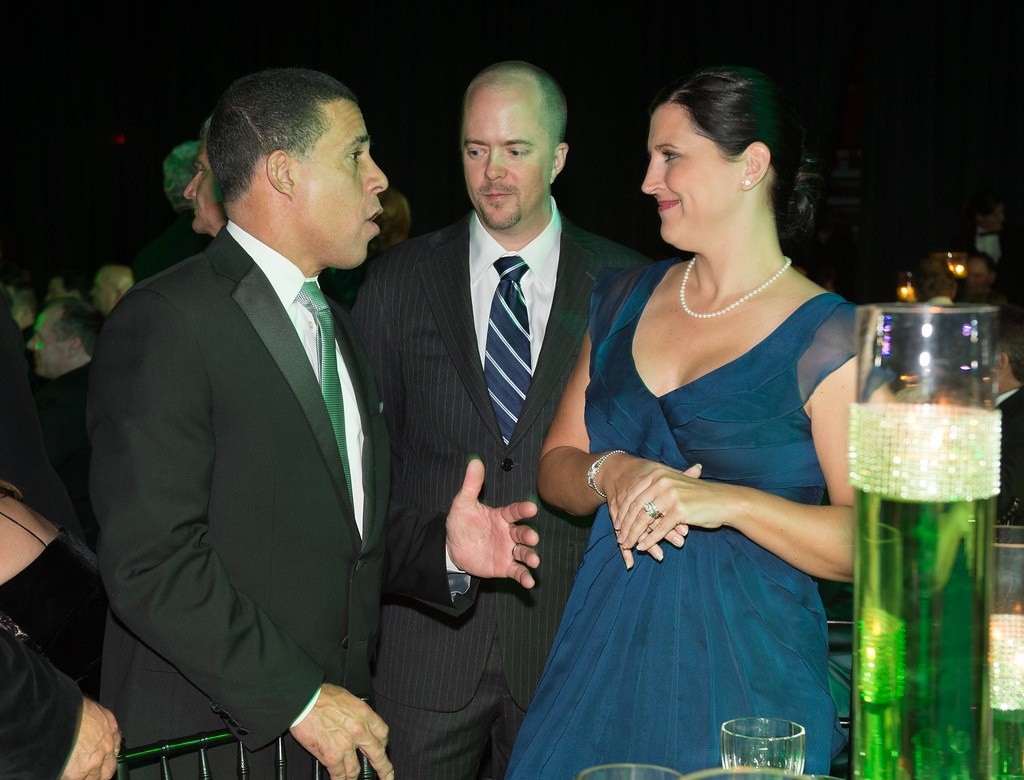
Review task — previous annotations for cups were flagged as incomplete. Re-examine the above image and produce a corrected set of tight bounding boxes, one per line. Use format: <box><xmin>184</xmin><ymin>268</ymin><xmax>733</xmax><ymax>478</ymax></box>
<box><xmin>719</xmin><ymin>717</ymin><xmax>806</xmax><ymax>777</ymax></box>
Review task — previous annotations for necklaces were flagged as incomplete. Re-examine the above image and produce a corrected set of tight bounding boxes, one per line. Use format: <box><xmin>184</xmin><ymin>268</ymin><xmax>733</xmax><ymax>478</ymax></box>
<box><xmin>679</xmin><ymin>251</ymin><xmax>792</xmax><ymax>318</ymax></box>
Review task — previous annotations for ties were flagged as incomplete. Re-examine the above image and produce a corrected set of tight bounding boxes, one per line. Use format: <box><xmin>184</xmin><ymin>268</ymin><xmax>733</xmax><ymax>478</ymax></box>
<box><xmin>484</xmin><ymin>256</ymin><xmax>532</xmax><ymax>450</ymax></box>
<box><xmin>294</xmin><ymin>281</ymin><xmax>353</xmax><ymax>523</ymax></box>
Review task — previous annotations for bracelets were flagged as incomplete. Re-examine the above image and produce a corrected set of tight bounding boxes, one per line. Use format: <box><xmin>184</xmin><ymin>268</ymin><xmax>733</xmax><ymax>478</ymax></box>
<box><xmin>588</xmin><ymin>450</ymin><xmax>628</xmax><ymax>498</ymax></box>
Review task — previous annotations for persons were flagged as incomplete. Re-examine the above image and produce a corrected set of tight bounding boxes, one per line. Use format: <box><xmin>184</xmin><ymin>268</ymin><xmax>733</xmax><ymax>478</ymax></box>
<box><xmin>793</xmin><ymin>207</ymin><xmax>1024</xmax><ymax>528</ymax></box>
<box><xmin>182</xmin><ymin>113</ymin><xmax>228</xmax><ymax>238</ymax></box>
<box><xmin>133</xmin><ymin>140</ymin><xmax>215</xmax><ymax>284</ymax></box>
<box><xmin>343</xmin><ymin>64</ymin><xmax>659</xmax><ymax>780</ymax></box>
<box><xmin>335</xmin><ymin>184</ymin><xmax>411</xmax><ymax>307</ymax></box>
<box><xmin>0</xmin><ymin>260</ymin><xmax>140</xmax><ymax>780</ymax></box>
<box><xmin>84</xmin><ymin>66</ymin><xmax>541</xmax><ymax>780</ymax></box>
<box><xmin>503</xmin><ymin>61</ymin><xmax>895</xmax><ymax>780</ymax></box>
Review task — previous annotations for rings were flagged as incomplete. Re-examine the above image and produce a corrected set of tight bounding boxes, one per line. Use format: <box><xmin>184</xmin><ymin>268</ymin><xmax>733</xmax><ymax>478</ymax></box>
<box><xmin>512</xmin><ymin>543</ymin><xmax>521</xmax><ymax>557</ymax></box>
<box><xmin>641</xmin><ymin>502</ymin><xmax>661</xmax><ymax>519</ymax></box>
